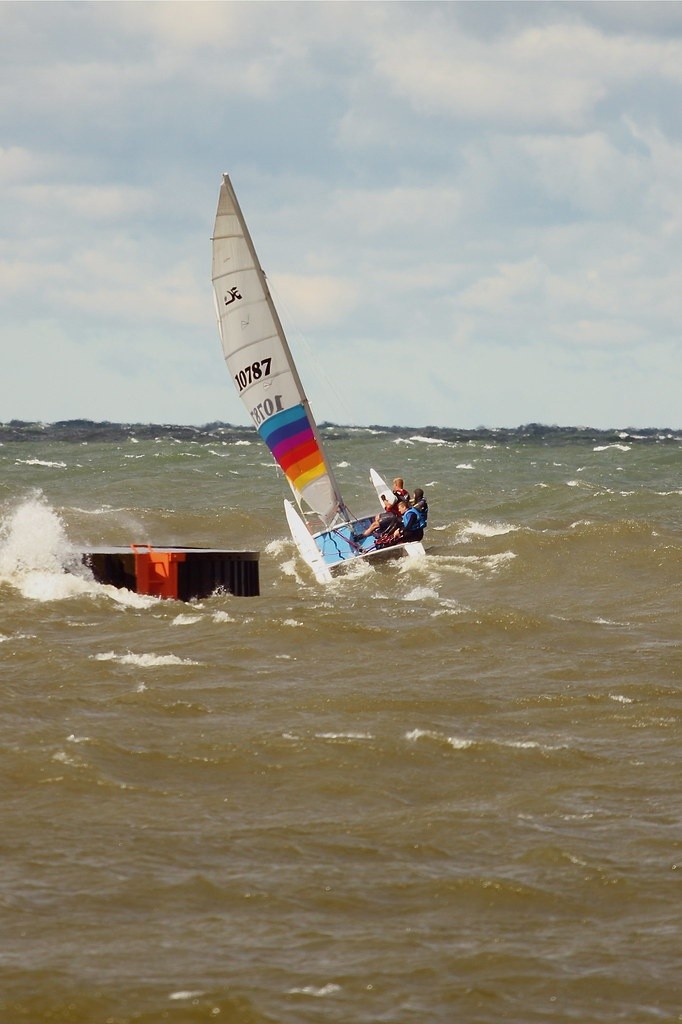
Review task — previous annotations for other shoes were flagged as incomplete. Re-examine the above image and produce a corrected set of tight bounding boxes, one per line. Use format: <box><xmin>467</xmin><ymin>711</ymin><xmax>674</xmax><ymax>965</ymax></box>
<box><xmin>353</xmin><ymin>534</ymin><xmax>364</xmax><ymax>540</ymax></box>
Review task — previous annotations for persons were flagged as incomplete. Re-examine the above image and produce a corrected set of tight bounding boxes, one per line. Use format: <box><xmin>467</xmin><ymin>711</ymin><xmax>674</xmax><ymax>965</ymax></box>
<box><xmin>353</xmin><ymin>478</ymin><xmax>410</xmax><ymax>542</ymax></box>
<box><xmin>393</xmin><ymin>501</ymin><xmax>427</xmax><ymax>544</ymax></box>
<box><xmin>382</xmin><ymin>488</ymin><xmax>429</xmax><ymax>537</ymax></box>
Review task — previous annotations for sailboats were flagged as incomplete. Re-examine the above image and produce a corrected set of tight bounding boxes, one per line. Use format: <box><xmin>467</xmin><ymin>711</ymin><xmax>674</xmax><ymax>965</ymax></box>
<box><xmin>208</xmin><ymin>173</ymin><xmax>427</xmax><ymax>584</ymax></box>
